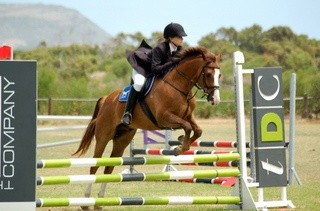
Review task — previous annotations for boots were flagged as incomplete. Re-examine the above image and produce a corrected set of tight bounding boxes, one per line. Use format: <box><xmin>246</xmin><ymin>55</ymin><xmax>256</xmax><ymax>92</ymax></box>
<box><xmin>121</xmin><ymin>88</ymin><xmax>142</xmax><ymax>125</ymax></box>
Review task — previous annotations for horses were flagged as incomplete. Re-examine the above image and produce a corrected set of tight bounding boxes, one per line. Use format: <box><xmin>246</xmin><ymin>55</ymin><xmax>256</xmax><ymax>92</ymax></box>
<box><xmin>71</xmin><ymin>47</ymin><xmax>221</xmax><ymax>210</ymax></box>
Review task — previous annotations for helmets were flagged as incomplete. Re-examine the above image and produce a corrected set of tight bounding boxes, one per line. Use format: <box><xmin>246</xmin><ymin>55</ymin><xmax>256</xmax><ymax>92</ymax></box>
<box><xmin>164</xmin><ymin>23</ymin><xmax>187</xmax><ymax>36</ymax></box>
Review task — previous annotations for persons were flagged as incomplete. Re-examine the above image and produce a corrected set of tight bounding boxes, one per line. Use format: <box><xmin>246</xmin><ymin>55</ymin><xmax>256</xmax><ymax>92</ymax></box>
<box><xmin>118</xmin><ymin>23</ymin><xmax>189</xmax><ymax>128</ymax></box>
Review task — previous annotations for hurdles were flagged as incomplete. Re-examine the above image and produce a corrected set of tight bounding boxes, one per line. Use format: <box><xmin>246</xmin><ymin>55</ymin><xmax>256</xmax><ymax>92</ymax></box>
<box><xmin>1</xmin><ymin>49</ymin><xmax>298</xmax><ymax>207</ymax></box>
